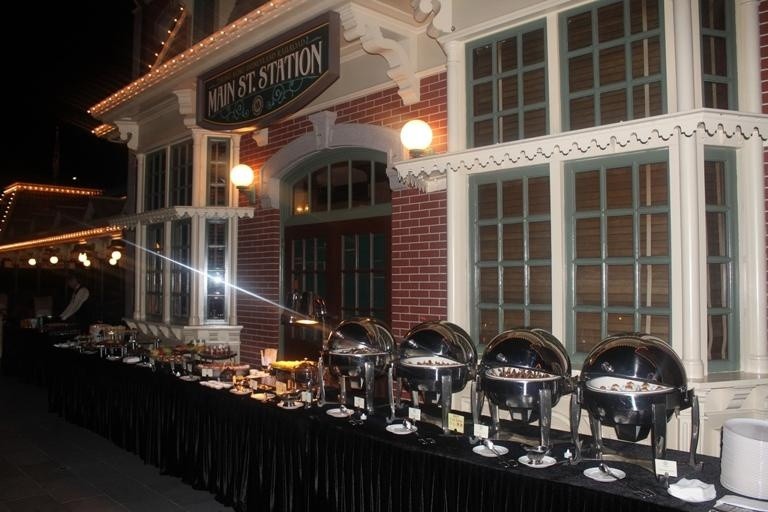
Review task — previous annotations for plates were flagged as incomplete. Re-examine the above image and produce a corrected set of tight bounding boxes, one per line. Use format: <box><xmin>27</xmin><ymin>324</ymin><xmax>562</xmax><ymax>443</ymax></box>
<box><xmin>107</xmin><ymin>355</ymin><xmax>120</xmax><ymax>361</ymax></box>
<box><xmin>230</xmin><ymin>388</ymin><xmax>304</xmax><ymax>409</ymax></box>
<box><xmin>386</xmin><ymin>423</ymin><xmax>417</xmax><ymax>436</ymax></box>
<box><xmin>719</xmin><ymin>417</ymin><xmax>768</xmax><ymax>501</ymax></box>
<box><xmin>180</xmin><ymin>374</ymin><xmax>199</xmax><ymax>382</ymax></box>
<box><xmin>326</xmin><ymin>408</ymin><xmax>355</xmax><ymax>417</ymax></box>
<box><xmin>473</xmin><ymin>445</ymin><xmax>717</xmax><ymax>502</ymax></box>
<box><xmin>124</xmin><ymin>356</ymin><xmax>140</xmax><ymax>364</ymax></box>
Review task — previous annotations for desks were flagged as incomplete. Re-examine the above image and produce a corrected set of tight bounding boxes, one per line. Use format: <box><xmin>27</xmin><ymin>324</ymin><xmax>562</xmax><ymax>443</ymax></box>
<box><xmin>14</xmin><ymin>318</ymin><xmax>768</xmax><ymax>512</ymax></box>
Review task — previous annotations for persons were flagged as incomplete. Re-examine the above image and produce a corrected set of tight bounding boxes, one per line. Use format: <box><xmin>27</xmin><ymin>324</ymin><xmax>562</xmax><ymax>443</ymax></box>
<box><xmin>59</xmin><ymin>273</ymin><xmax>91</xmax><ymax>321</ymax></box>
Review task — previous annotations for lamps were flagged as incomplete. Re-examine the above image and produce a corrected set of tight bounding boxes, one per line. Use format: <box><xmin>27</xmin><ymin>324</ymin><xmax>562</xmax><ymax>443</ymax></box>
<box><xmin>229</xmin><ymin>164</ymin><xmax>258</xmax><ymax>205</ymax></box>
<box><xmin>397</xmin><ymin>119</ymin><xmax>436</xmax><ymax>159</ymax></box>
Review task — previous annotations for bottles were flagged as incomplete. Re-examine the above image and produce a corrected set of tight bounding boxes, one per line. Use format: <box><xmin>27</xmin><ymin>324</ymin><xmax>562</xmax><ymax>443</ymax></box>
<box><xmin>301</xmin><ymin>369</ymin><xmax>312</xmax><ymax>409</ymax></box>
